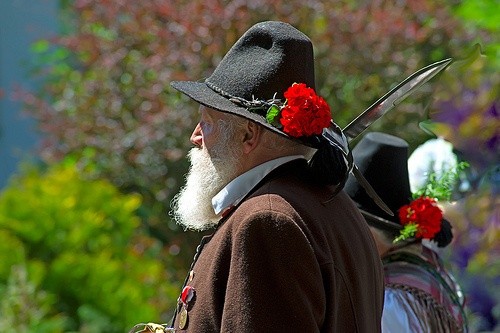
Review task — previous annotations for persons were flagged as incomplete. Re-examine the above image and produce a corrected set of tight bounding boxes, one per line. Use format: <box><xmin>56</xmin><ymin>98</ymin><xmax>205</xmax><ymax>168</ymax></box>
<box><xmin>127</xmin><ymin>21</ymin><xmax>387</xmax><ymax>333</ymax></box>
<box><xmin>343</xmin><ymin>126</ymin><xmax>469</xmax><ymax>332</ymax></box>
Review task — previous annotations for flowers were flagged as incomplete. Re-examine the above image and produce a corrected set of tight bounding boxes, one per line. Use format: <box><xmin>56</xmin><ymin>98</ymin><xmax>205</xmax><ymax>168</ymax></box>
<box><xmin>392</xmin><ymin>162</ymin><xmax>471</xmax><ymax>248</ymax></box>
<box><xmin>267</xmin><ymin>83</ymin><xmax>330</xmax><ymax>138</ymax></box>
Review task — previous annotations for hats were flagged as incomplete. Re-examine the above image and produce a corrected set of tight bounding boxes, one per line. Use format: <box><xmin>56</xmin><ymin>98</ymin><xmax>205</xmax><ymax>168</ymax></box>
<box><xmin>346</xmin><ymin>134</ymin><xmax>443</xmax><ymax>241</ymax></box>
<box><xmin>169</xmin><ymin>19</ymin><xmax>332</xmax><ymax>151</ymax></box>
